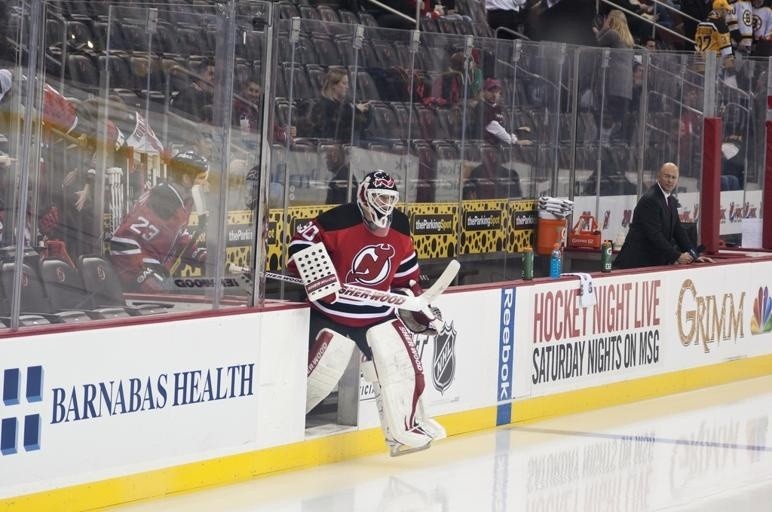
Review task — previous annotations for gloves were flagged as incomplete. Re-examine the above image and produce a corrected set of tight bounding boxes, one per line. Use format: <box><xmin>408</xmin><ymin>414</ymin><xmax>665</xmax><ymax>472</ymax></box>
<box><xmin>137</xmin><ymin>267</ymin><xmax>170</xmax><ymax>293</ymax></box>
<box><xmin>194</xmin><ymin>246</ymin><xmax>208</xmax><ymax>261</ymax></box>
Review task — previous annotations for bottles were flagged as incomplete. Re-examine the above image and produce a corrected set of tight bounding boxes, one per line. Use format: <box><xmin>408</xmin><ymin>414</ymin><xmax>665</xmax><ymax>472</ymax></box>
<box><xmin>521</xmin><ymin>247</ymin><xmax>535</xmax><ymax>280</ymax></box>
<box><xmin>601</xmin><ymin>240</ymin><xmax>613</xmax><ymax>272</ymax></box>
<box><xmin>550</xmin><ymin>242</ymin><xmax>562</xmax><ymax>279</ymax></box>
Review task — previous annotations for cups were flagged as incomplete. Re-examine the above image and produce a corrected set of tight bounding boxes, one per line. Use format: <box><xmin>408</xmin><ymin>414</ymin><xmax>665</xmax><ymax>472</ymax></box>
<box><xmin>240</xmin><ymin>120</ymin><xmax>249</xmax><ymax>135</ymax></box>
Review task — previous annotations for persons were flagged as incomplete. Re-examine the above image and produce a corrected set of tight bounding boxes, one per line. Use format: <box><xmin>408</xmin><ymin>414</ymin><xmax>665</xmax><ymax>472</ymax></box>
<box><xmin>2</xmin><ymin>1</ymin><xmax>772</xmax><ymax>294</ymax></box>
<box><xmin>288</xmin><ymin>169</ymin><xmax>446</xmax><ymax>448</ymax></box>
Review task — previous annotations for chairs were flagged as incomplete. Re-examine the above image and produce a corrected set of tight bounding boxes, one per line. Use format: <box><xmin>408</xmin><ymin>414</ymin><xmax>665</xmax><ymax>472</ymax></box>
<box><xmin>0</xmin><ymin>0</ymin><xmax>771</xmax><ymax>203</ymax></box>
<box><xmin>0</xmin><ymin>258</ymin><xmax>168</xmax><ymax>330</ymax></box>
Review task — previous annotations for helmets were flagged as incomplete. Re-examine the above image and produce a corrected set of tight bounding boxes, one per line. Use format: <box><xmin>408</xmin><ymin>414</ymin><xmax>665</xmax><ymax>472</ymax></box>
<box><xmin>171</xmin><ymin>152</ymin><xmax>208</xmax><ymax>187</ymax></box>
<box><xmin>357</xmin><ymin>170</ymin><xmax>399</xmax><ymax>228</ymax></box>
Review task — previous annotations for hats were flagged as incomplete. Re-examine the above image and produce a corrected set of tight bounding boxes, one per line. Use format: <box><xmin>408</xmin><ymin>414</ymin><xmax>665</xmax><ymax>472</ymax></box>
<box><xmin>484</xmin><ymin>78</ymin><xmax>500</xmax><ymax>90</ymax></box>
<box><xmin>713</xmin><ymin>0</ymin><xmax>733</xmax><ymax>11</ymax></box>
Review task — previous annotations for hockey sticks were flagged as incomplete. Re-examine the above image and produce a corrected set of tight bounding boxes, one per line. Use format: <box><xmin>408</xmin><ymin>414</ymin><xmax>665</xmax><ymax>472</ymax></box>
<box><xmin>167</xmin><ymin>184</ymin><xmax>208</xmax><ymax>280</ymax></box>
<box><xmin>227</xmin><ymin>260</ymin><xmax>460</xmax><ymax>312</ymax></box>
